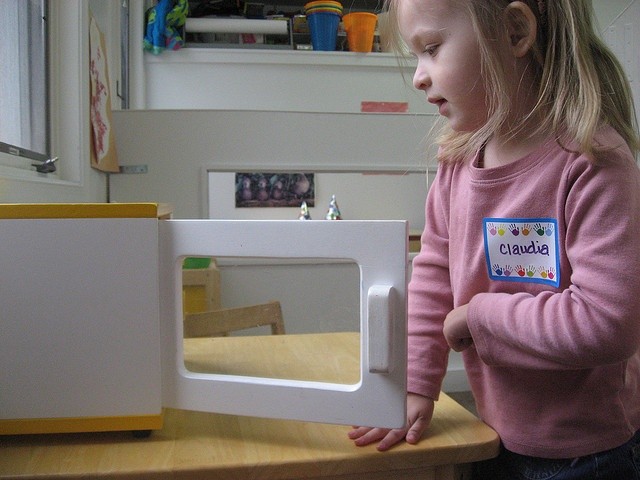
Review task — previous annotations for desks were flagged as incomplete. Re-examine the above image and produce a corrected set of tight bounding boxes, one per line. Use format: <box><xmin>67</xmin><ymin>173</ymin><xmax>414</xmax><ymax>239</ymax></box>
<box><xmin>1</xmin><ymin>331</ymin><xmax>501</xmax><ymax>480</ymax></box>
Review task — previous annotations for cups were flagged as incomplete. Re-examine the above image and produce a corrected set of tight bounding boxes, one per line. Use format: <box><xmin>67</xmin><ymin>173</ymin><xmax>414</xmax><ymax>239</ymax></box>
<box><xmin>304</xmin><ymin>1</ymin><xmax>343</xmax><ymax>52</ymax></box>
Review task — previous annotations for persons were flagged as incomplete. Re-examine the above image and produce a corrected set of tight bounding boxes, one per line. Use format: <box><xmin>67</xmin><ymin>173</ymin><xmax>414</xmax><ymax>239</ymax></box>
<box><xmin>346</xmin><ymin>0</ymin><xmax>640</xmax><ymax>479</ymax></box>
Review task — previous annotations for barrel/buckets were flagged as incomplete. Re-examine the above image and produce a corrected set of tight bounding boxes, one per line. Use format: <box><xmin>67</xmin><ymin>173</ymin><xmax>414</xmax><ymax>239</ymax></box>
<box><xmin>304</xmin><ymin>0</ymin><xmax>343</xmax><ymax>50</ymax></box>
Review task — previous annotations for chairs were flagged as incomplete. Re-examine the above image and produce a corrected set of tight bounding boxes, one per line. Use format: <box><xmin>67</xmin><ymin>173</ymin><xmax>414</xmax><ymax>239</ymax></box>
<box><xmin>181</xmin><ymin>269</ymin><xmax>230</xmax><ymax>336</ymax></box>
<box><xmin>185</xmin><ymin>300</ymin><xmax>286</xmax><ymax>337</ymax></box>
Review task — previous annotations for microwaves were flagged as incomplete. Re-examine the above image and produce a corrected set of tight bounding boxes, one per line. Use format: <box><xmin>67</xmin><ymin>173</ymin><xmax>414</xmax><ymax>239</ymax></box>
<box><xmin>0</xmin><ymin>193</ymin><xmax>409</xmax><ymax>446</ymax></box>
